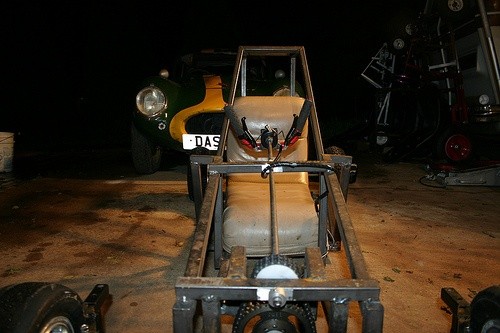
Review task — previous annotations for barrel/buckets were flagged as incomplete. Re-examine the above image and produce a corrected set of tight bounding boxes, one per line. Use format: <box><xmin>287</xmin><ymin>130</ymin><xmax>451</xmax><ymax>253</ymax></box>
<box><xmin>0</xmin><ymin>132</ymin><xmax>15</xmax><ymax>174</ymax></box>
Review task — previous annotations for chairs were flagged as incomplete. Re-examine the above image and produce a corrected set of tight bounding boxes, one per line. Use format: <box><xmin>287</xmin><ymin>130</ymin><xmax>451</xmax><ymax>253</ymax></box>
<box><xmin>222</xmin><ymin>96</ymin><xmax>318</xmax><ymax>258</ymax></box>
